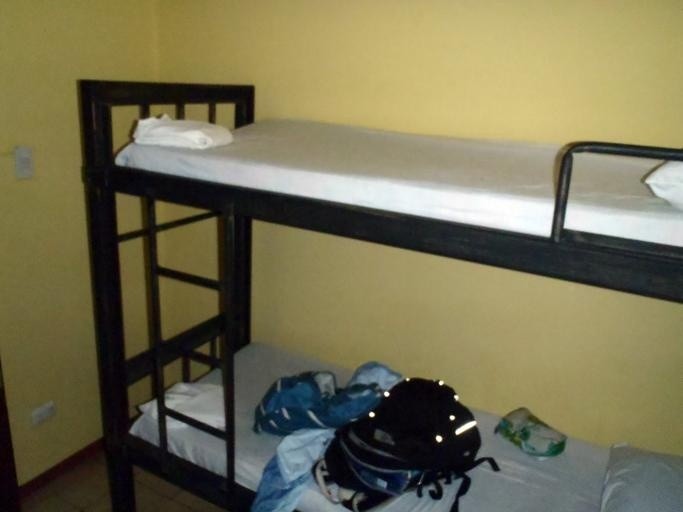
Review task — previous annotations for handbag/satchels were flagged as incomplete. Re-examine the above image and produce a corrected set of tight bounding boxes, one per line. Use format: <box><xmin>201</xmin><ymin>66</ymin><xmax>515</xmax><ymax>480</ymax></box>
<box><xmin>255</xmin><ymin>371</ymin><xmax>383</xmax><ymax>436</ymax></box>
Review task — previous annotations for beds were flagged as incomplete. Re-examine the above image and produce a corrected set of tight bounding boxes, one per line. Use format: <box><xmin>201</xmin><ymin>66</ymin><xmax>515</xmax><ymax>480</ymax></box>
<box><xmin>76</xmin><ymin>79</ymin><xmax>683</xmax><ymax>512</ymax></box>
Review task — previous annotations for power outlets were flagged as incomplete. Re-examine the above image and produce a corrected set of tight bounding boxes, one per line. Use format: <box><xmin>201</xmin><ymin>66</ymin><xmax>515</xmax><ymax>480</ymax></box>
<box><xmin>32</xmin><ymin>401</ymin><xmax>58</xmax><ymax>427</ymax></box>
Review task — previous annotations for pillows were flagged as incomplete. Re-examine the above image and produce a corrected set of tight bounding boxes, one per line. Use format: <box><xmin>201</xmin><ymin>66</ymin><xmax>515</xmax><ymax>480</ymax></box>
<box><xmin>641</xmin><ymin>158</ymin><xmax>683</xmax><ymax>211</ymax></box>
<box><xmin>600</xmin><ymin>441</ymin><xmax>683</xmax><ymax>512</ymax></box>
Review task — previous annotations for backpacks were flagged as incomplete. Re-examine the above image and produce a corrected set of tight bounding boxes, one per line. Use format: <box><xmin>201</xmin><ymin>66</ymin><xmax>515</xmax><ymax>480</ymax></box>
<box><xmin>312</xmin><ymin>378</ymin><xmax>479</xmax><ymax>511</ymax></box>
